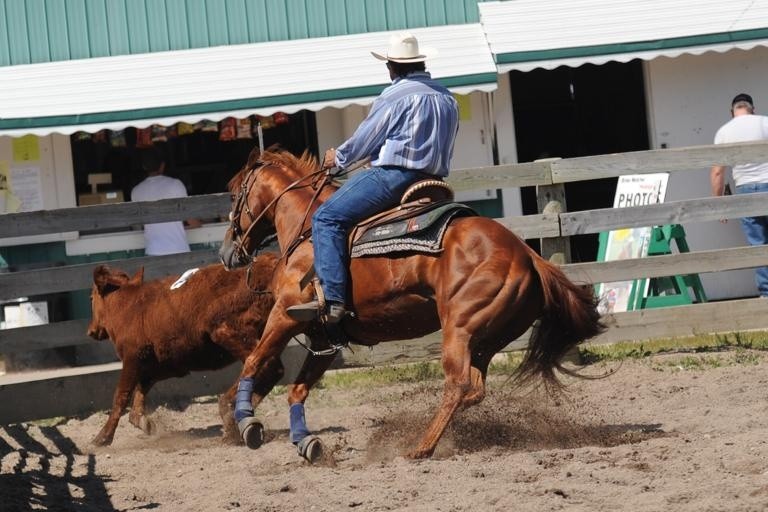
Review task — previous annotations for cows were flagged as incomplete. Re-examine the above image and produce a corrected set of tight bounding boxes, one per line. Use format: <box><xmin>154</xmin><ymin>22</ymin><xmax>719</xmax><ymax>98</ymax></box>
<box><xmin>84</xmin><ymin>252</ymin><xmax>289</xmax><ymax>448</ymax></box>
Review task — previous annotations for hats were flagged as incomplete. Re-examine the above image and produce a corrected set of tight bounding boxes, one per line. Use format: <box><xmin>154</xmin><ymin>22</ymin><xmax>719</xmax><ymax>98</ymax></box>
<box><xmin>731</xmin><ymin>94</ymin><xmax>754</xmax><ymax>106</ymax></box>
<box><xmin>370</xmin><ymin>34</ymin><xmax>434</xmax><ymax>64</ymax></box>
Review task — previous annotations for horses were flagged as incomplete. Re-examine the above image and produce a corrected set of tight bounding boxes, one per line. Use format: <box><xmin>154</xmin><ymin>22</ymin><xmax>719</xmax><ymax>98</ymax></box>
<box><xmin>216</xmin><ymin>138</ymin><xmax>621</xmax><ymax>463</ymax></box>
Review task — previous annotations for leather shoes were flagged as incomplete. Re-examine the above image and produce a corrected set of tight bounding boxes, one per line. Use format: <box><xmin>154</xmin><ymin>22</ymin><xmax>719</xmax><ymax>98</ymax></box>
<box><xmin>284</xmin><ymin>298</ymin><xmax>346</xmax><ymax>325</ymax></box>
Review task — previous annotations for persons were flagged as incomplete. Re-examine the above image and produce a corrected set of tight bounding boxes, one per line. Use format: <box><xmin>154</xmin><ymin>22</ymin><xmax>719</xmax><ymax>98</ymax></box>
<box><xmin>283</xmin><ymin>30</ymin><xmax>464</xmax><ymax>324</ymax></box>
<box><xmin>712</xmin><ymin>92</ymin><xmax>767</xmax><ymax>295</ymax></box>
<box><xmin>127</xmin><ymin>148</ymin><xmax>208</xmax><ymax>258</ymax></box>
